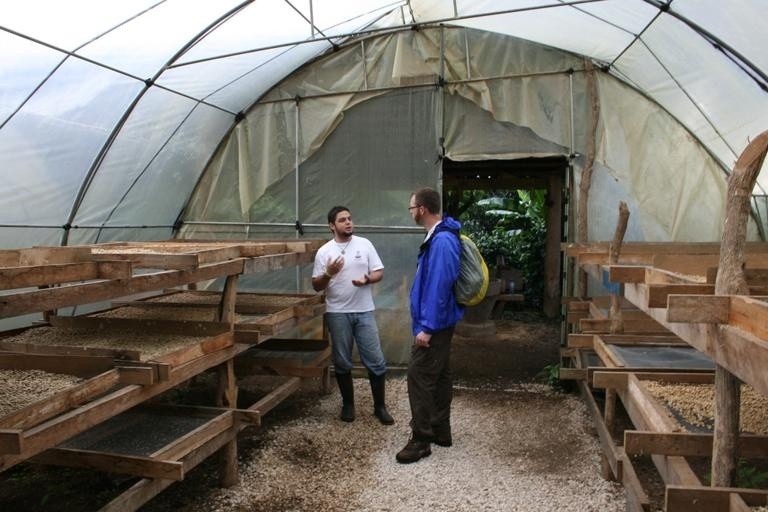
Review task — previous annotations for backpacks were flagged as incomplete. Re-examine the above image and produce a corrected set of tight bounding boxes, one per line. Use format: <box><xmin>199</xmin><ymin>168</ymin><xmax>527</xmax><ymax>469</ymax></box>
<box><xmin>425</xmin><ymin>228</ymin><xmax>489</xmax><ymax>306</ymax></box>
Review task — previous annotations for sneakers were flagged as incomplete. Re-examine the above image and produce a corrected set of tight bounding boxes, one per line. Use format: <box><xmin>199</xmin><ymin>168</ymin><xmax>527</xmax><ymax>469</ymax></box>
<box><xmin>396</xmin><ymin>439</ymin><xmax>430</xmax><ymax>462</ymax></box>
<box><xmin>431</xmin><ymin>437</ymin><xmax>452</xmax><ymax>446</ymax></box>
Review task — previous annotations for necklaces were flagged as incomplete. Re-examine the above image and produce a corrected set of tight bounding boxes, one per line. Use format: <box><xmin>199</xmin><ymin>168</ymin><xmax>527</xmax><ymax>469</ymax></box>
<box><xmin>336</xmin><ymin>237</ymin><xmax>352</xmax><ymax>254</ymax></box>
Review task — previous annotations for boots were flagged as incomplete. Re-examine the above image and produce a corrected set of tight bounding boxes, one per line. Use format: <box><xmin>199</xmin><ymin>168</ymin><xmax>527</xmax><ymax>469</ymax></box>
<box><xmin>335</xmin><ymin>371</ymin><xmax>354</xmax><ymax>422</ymax></box>
<box><xmin>369</xmin><ymin>372</ymin><xmax>394</xmax><ymax>425</ymax></box>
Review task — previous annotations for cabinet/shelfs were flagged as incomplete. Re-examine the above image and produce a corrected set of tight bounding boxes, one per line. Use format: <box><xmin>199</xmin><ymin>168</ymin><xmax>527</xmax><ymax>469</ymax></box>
<box><xmin>558</xmin><ymin>239</ymin><xmax>767</xmax><ymax>511</ymax></box>
<box><xmin>1</xmin><ymin>237</ymin><xmax>334</xmax><ymax>512</ymax></box>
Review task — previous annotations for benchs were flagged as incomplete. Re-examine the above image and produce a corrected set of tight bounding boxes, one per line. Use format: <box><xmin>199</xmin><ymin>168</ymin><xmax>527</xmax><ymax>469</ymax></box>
<box><xmin>489</xmin><ymin>271</ymin><xmax>524</xmax><ymax>319</ymax></box>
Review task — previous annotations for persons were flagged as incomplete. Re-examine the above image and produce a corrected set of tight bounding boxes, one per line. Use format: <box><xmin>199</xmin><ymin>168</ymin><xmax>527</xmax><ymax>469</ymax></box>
<box><xmin>313</xmin><ymin>206</ymin><xmax>395</xmax><ymax>425</ymax></box>
<box><xmin>397</xmin><ymin>189</ymin><xmax>466</xmax><ymax>463</ymax></box>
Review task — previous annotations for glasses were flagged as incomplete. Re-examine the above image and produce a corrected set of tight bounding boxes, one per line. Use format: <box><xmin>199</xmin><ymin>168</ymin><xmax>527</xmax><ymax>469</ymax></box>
<box><xmin>408</xmin><ymin>206</ymin><xmax>418</xmax><ymax>211</ymax></box>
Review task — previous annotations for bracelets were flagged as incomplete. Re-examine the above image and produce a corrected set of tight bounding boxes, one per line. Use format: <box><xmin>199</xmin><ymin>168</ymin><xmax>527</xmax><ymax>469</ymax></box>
<box><xmin>324</xmin><ymin>271</ymin><xmax>331</xmax><ymax>279</ymax></box>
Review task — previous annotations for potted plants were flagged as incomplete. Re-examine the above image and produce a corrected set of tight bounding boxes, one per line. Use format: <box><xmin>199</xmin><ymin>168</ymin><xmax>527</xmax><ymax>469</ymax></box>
<box><xmin>454</xmin><ymin>233</ymin><xmax>501</xmax><ymax>338</ymax></box>
